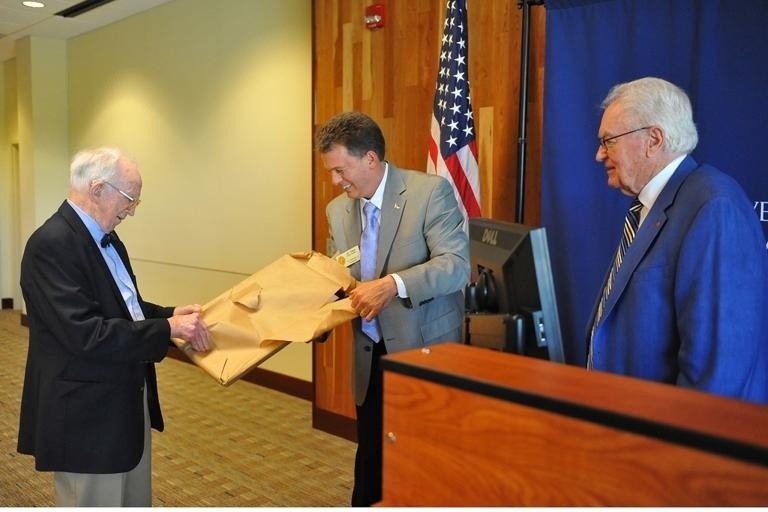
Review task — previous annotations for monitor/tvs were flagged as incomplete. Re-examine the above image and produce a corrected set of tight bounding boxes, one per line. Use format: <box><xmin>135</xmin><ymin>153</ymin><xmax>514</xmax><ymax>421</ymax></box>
<box><xmin>464</xmin><ymin>218</ymin><xmax>566</xmax><ymax>364</ymax></box>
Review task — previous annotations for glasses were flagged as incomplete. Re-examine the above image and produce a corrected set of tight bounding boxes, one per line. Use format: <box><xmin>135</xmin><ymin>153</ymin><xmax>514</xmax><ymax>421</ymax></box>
<box><xmin>104</xmin><ymin>181</ymin><xmax>141</xmax><ymax>208</ymax></box>
<box><xmin>600</xmin><ymin>127</ymin><xmax>647</xmax><ymax>151</ymax></box>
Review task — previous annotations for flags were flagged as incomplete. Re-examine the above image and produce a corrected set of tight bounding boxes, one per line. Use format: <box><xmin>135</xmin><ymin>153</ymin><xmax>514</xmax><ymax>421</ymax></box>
<box><xmin>426</xmin><ymin>0</ymin><xmax>482</xmax><ymax>221</ymax></box>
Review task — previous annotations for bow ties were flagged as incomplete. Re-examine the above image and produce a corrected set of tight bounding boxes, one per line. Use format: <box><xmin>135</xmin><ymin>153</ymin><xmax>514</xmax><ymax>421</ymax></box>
<box><xmin>100</xmin><ymin>232</ymin><xmax>117</xmax><ymax>248</ymax></box>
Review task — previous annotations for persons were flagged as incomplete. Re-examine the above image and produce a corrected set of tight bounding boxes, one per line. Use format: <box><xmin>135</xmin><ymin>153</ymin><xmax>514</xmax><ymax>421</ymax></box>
<box><xmin>16</xmin><ymin>147</ymin><xmax>218</xmax><ymax>507</ymax></box>
<box><xmin>317</xmin><ymin>111</ymin><xmax>472</xmax><ymax>506</ymax></box>
<box><xmin>583</xmin><ymin>77</ymin><xmax>768</xmax><ymax>405</ymax></box>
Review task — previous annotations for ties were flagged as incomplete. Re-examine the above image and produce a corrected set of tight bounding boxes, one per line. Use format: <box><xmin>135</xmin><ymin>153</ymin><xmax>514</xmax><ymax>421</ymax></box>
<box><xmin>359</xmin><ymin>202</ymin><xmax>382</xmax><ymax>344</ymax></box>
<box><xmin>586</xmin><ymin>197</ymin><xmax>642</xmax><ymax>369</ymax></box>
